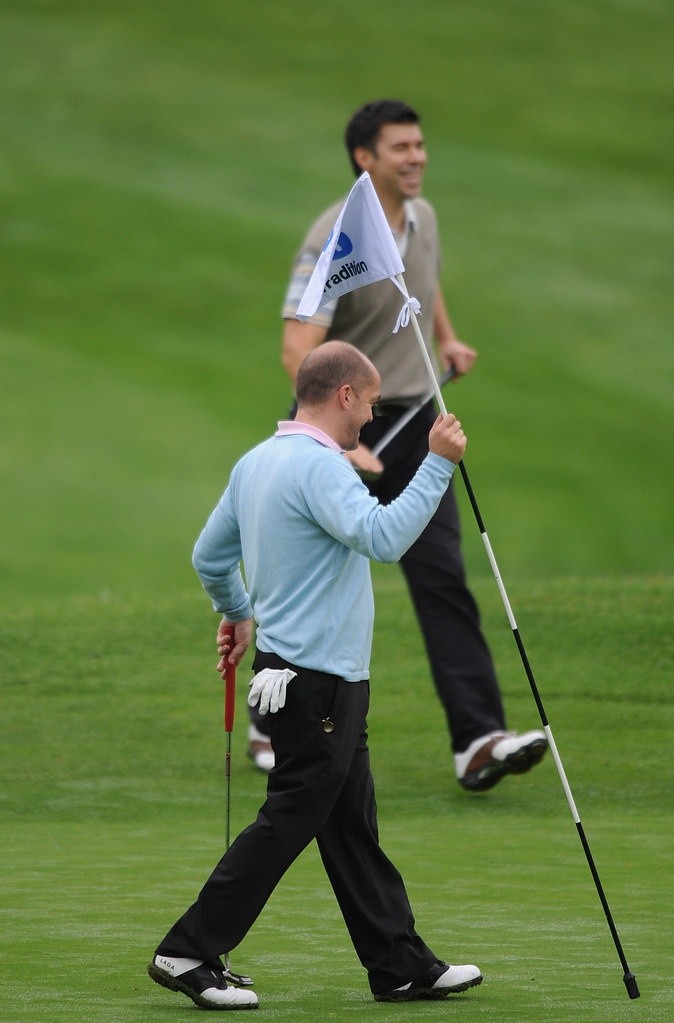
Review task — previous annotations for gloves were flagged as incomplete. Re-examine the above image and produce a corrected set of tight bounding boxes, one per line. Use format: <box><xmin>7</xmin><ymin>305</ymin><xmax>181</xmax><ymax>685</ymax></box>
<box><xmin>246</xmin><ymin>668</ymin><xmax>297</xmax><ymax>716</ymax></box>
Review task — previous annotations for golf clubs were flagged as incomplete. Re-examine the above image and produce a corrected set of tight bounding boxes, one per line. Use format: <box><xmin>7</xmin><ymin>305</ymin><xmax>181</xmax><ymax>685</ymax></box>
<box><xmin>372</xmin><ymin>366</ymin><xmax>457</xmax><ymax>456</ymax></box>
<box><xmin>223</xmin><ymin>626</ymin><xmax>255</xmax><ymax>987</ymax></box>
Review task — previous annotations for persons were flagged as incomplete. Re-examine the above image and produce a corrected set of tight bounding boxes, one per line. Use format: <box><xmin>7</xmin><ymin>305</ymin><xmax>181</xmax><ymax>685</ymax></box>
<box><xmin>139</xmin><ymin>340</ymin><xmax>483</xmax><ymax>1004</ymax></box>
<box><xmin>245</xmin><ymin>98</ymin><xmax>553</xmax><ymax>793</ymax></box>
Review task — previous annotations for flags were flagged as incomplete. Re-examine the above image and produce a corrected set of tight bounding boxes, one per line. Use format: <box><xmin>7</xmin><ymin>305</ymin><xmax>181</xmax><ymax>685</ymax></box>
<box><xmin>297</xmin><ymin>173</ymin><xmax>409</xmax><ymax>325</ymax></box>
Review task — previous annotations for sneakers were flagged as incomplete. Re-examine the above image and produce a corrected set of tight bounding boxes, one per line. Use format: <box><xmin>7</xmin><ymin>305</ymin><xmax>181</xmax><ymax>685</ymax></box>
<box><xmin>146</xmin><ymin>953</ymin><xmax>260</xmax><ymax>1010</ymax></box>
<box><xmin>455</xmin><ymin>727</ymin><xmax>548</xmax><ymax>793</ymax></box>
<box><xmin>373</xmin><ymin>956</ymin><xmax>482</xmax><ymax>1002</ymax></box>
<box><xmin>245</xmin><ymin>722</ymin><xmax>276</xmax><ymax>771</ymax></box>
